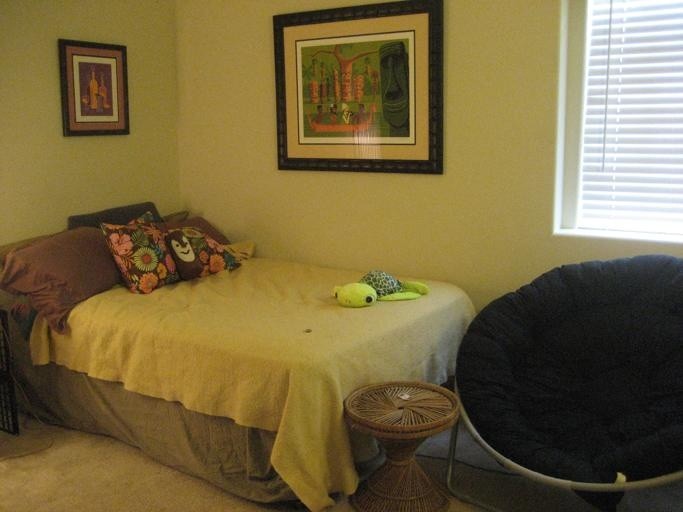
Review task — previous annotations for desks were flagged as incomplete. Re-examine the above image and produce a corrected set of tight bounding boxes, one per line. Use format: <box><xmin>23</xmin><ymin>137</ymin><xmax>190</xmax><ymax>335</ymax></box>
<box><xmin>345</xmin><ymin>381</ymin><xmax>460</xmax><ymax>512</ymax></box>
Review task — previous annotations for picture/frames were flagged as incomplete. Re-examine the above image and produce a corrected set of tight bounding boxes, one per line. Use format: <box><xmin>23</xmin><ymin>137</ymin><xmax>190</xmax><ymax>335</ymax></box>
<box><xmin>271</xmin><ymin>1</ymin><xmax>444</xmax><ymax>174</ymax></box>
<box><xmin>56</xmin><ymin>38</ymin><xmax>130</xmax><ymax>136</ymax></box>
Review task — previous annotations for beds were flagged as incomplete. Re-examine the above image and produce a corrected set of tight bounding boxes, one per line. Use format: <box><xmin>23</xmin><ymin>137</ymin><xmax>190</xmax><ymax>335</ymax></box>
<box><xmin>1</xmin><ymin>212</ymin><xmax>478</xmax><ymax>508</ymax></box>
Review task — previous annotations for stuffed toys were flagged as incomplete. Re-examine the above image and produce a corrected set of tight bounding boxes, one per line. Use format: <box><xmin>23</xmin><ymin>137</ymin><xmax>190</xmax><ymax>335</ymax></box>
<box><xmin>332</xmin><ymin>269</ymin><xmax>429</xmax><ymax>308</ymax></box>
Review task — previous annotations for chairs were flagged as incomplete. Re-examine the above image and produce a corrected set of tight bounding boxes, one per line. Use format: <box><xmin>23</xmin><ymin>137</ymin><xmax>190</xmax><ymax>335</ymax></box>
<box><xmin>447</xmin><ymin>256</ymin><xmax>683</xmax><ymax>509</ymax></box>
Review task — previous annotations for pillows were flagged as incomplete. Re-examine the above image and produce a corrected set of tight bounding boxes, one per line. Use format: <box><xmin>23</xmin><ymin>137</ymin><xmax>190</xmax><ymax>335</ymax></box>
<box><xmin>2</xmin><ymin>201</ymin><xmax>243</xmax><ymax>337</ymax></box>
